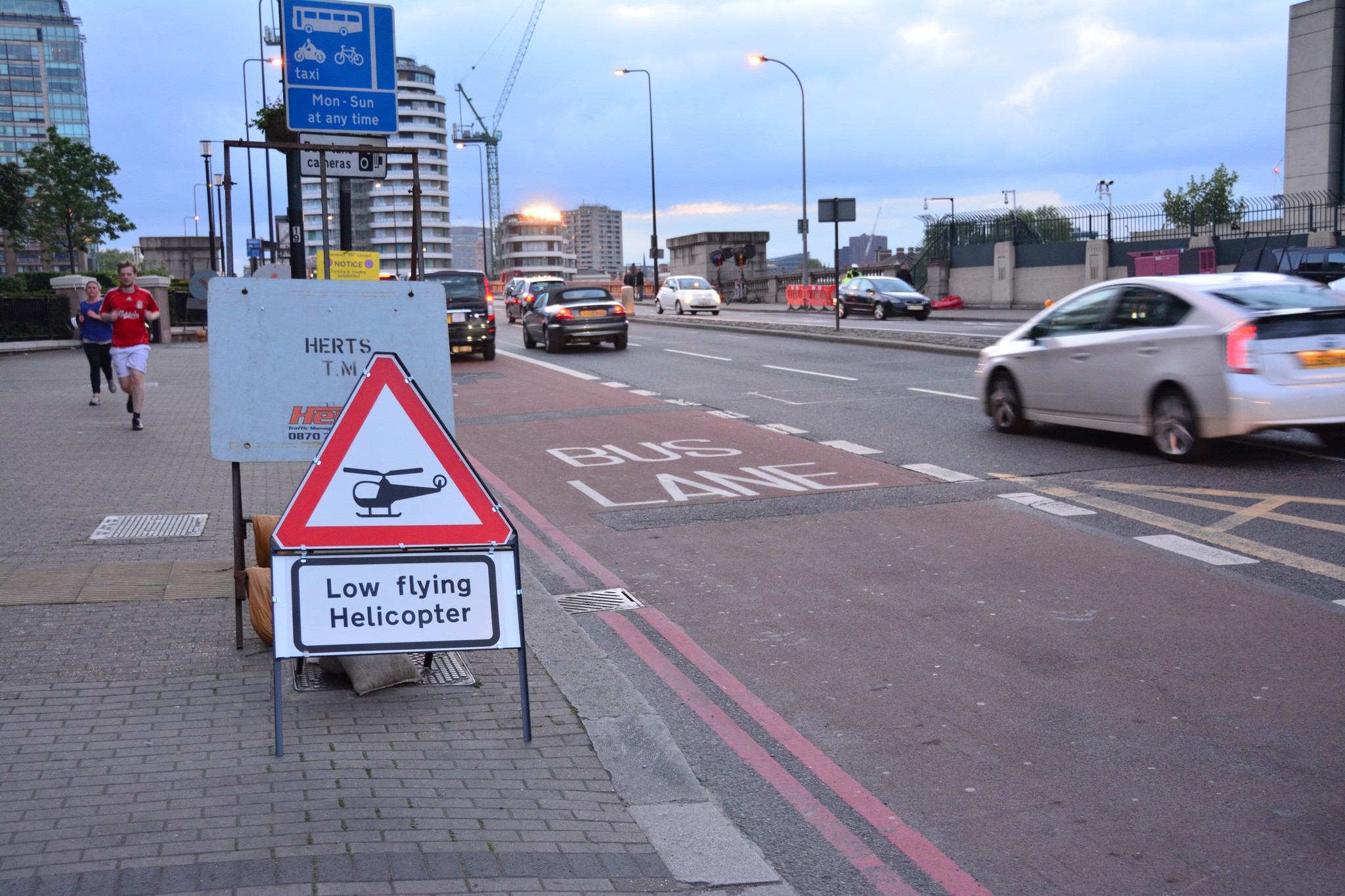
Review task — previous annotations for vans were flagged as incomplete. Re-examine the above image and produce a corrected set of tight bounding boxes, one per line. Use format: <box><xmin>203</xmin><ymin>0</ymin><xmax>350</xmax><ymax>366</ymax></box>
<box><xmin>398</xmin><ymin>268</ymin><xmax>496</xmax><ymax>361</ymax></box>
<box><xmin>380</xmin><ymin>272</ymin><xmax>400</xmax><ymax>281</ymax></box>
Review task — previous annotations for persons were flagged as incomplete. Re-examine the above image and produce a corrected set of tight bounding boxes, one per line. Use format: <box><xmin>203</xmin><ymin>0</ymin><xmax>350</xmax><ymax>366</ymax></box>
<box><xmin>624</xmin><ymin>270</ymin><xmax>635</xmax><ymax>300</ymax></box>
<box><xmin>842</xmin><ymin>263</ymin><xmax>863</xmax><ymax>284</ymax></box>
<box><xmin>99</xmin><ymin>260</ymin><xmax>160</xmax><ymax>430</ymax></box>
<box><xmin>75</xmin><ymin>280</ymin><xmax>117</xmax><ymax>406</ymax></box>
<box><xmin>635</xmin><ymin>268</ymin><xmax>644</xmax><ymax>302</ymax></box>
<box><xmin>895</xmin><ymin>263</ymin><xmax>912</xmax><ymax>286</ymax></box>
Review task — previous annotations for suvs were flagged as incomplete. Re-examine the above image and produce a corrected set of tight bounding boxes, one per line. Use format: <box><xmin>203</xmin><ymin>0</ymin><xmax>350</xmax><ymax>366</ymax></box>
<box><xmin>1274</xmin><ymin>245</ymin><xmax>1345</xmax><ymax>285</ymax></box>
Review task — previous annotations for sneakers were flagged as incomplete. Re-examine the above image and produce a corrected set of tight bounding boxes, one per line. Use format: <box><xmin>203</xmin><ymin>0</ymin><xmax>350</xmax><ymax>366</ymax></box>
<box><xmin>127</xmin><ymin>395</ymin><xmax>133</xmax><ymax>412</ymax></box>
<box><xmin>90</xmin><ymin>394</ymin><xmax>101</xmax><ymax>404</ymax></box>
<box><xmin>107</xmin><ymin>378</ymin><xmax>116</xmax><ymax>393</ymax></box>
<box><xmin>132</xmin><ymin>418</ymin><xmax>143</xmax><ymax>430</ymax></box>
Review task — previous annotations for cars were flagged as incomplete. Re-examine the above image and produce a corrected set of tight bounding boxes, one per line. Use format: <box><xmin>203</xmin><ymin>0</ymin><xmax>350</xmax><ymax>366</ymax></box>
<box><xmin>504</xmin><ymin>277</ymin><xmax>543</xmax><ymax>300</ymax></box>
<box><xmin>505</xmin><ymin>276</ymin><xmax>567</xmax><ymax>325</ymax></box>
<box><xmin>975</xmin><ymin>271</ymin><xmax>1345</xmax><ymax>465</ymax></box>
<box><xmin>833</xmin><ymin>276</ymin><xmax>931</xmax><ymax>321</ymax></box>
<box><xmin>654</xmin><ymin>275</ymin><xmax>721</xmax><ymax>316</ymax></box>
<box><xmin>522</xmin><ymin>285</ymin><xmax>629</xmax><ymax>353</ymax></box>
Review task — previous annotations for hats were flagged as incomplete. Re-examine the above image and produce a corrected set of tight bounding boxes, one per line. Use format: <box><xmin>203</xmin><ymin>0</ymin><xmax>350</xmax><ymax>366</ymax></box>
<box><xmin>851</xmin><ymin>264</ymin><xmax>858</xmax><ymax>268</ymax></box>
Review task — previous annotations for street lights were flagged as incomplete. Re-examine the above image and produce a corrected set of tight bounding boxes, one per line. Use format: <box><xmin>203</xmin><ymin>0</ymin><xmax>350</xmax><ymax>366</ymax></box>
<box><xmin>213</xmin><ymin>173</ymin><xmax>226</xmax><ymax>275</ymax></box>
<box><xmin>199</xmin><ymin>139</ymin><xmax>217</xmax><ymax>273</ymax></box>
<box><xmin>923</xmin><ymin>197</ymin><xmax>954</xmax><ymax>224</ymax></box>
<box><xmin>1001</xmin><ymin>189</ymin><xmax>1017</xmax><ymax>225</ymax></box>
<box><xmin>194</xmin><ymin>183</ymin><xmax>212</xmax><ymax>237</ymax></box>
<box><xmin>614</xmin><ymin>68</ymin><xmax>658</xmax><ymax>298</ymax></box>
<box><xmin>243</xmin><ymin>58</ymin><xmax>282</xmax><ymax>272</ymax></box>
<box><xmin>748</xmin><ymin>55</ymin><xmax>809</xmax><ymax>310</ymax></box>
<box><xmin>184</xmin><ymin>215</ymin><xmax>199</xmax><ymax>237</ymax></box>
<box><xmin>374</xmin><ymin>183</ymin><xmax>398</xmax><ymax>276</ymax></box>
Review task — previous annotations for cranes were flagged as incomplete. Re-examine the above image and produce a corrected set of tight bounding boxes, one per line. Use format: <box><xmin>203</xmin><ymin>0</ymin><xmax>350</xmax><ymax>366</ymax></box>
<box><xmin>451</xmin><ymin>0</ymin><xmax>545</xmax><ymax>282</ymax></box>
